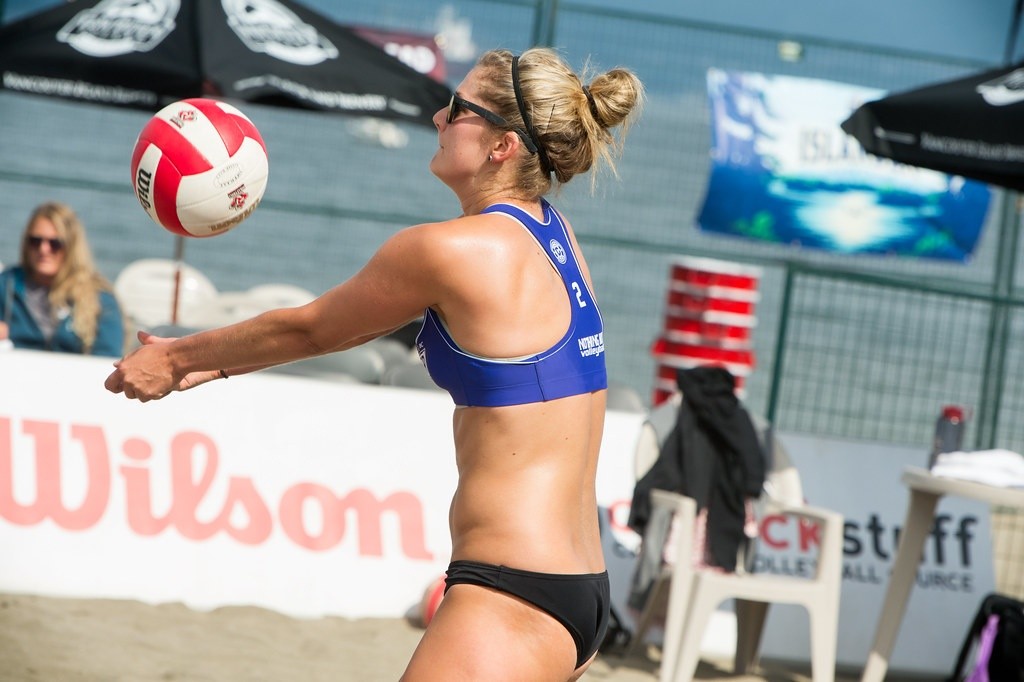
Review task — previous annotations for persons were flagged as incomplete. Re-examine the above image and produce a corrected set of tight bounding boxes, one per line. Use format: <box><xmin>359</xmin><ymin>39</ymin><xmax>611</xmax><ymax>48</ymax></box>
<box><xmin>104</xmin><ymin>46</ymin><xmax>647</xmax><ymax>682</ymax></box>
<box><xmin>0</xmin><ymin>203</ymin><xmax>127</xmax><ymax>358</ymax></box>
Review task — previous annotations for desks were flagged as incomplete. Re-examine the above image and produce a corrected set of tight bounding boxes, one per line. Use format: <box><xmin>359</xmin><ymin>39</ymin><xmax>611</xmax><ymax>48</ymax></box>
<box><xmin>858</xmin><ymin>463</ymin><xmax>1024</xmax><ymax>682</ymax></box>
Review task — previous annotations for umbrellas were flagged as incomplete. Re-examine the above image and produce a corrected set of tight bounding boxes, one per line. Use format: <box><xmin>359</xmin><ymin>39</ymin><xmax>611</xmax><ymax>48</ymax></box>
<box><xmin>0</xmin><ymin>0</ymin><xmax>454</xmax><ymax>334</ymax></box>
<box><xmin>840</xmin><ymin>56</ymin><xmax>1024</xmax><ymax>191</ymax></box>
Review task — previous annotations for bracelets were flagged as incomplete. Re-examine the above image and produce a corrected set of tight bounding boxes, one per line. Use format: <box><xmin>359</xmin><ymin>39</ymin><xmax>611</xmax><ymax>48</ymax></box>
<box><xmin>220</xmin><ymin>369</ymin><xmax>228</xmax><ymax>378</ymax></box>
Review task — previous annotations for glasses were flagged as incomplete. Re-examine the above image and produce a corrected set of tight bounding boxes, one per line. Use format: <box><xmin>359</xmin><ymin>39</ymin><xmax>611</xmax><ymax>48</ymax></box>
<box><xmin>27</xmin><ymin>233</ymin><xmax>63</xmax><ymax>253</ymax></box>
<box><xmin>446</xmin><ymin>93</ymin><xmax>540</xmax><ymax>156</ymax></box>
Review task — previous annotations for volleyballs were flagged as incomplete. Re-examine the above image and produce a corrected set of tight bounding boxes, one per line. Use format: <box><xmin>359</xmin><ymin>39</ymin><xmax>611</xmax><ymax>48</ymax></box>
<box><xmin>131</xmin><ymin>97</ymin><xmax>269</xmax><ymax>238</ymax></box>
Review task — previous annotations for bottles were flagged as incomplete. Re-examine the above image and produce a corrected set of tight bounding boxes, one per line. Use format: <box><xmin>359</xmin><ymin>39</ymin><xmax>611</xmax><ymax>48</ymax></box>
<box><xmin>929</xmin><ymin>405</ymin><xmax>973</xmax><ymax>470</ymax></box>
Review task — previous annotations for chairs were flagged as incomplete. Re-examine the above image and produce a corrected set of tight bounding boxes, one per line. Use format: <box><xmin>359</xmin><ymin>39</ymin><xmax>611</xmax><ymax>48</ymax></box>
<box><xmin>615</xmin><ymin>379</ymin><xmax>845</xmax><ymax>682</ymax></box>
